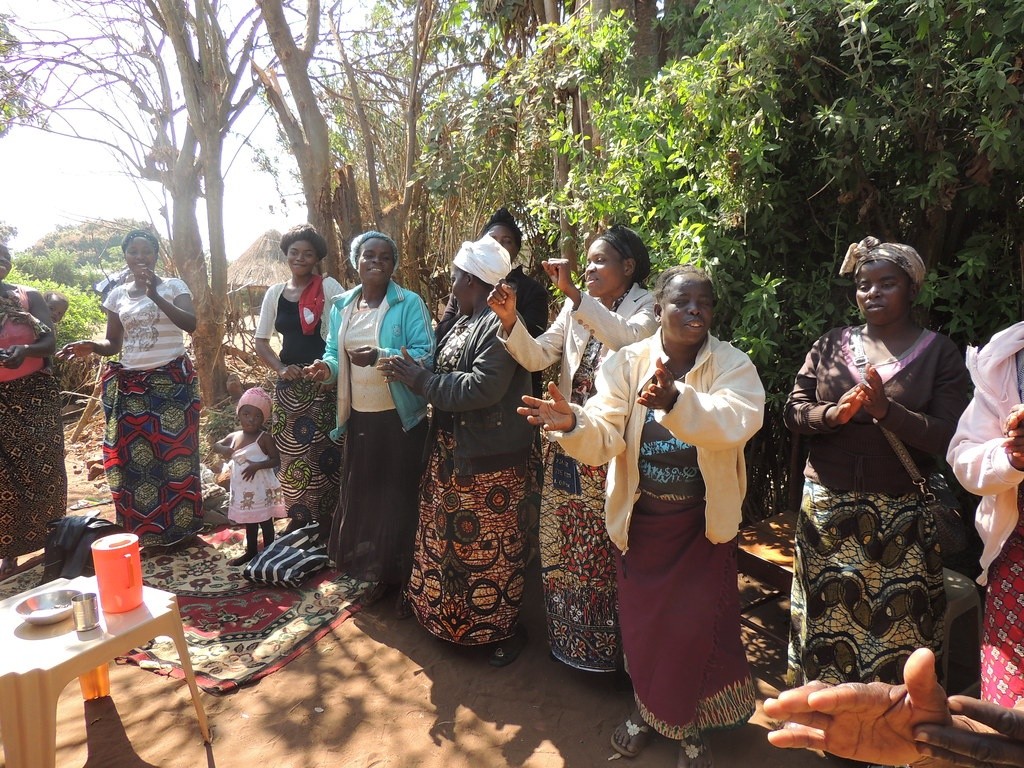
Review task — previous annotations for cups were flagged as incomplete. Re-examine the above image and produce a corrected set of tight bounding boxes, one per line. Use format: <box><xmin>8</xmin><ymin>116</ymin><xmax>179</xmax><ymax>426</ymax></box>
<box><xmin>71</xmin><ymin>592</ymin><xmax>99</xmax><ymax>632</ymax></box>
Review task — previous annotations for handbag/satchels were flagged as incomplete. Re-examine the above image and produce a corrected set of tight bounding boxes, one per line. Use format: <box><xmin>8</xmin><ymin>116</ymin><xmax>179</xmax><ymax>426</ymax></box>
<box><xmin>241</xmin><ymin>521</ymin><xmax>331</xmax><ymax>589</ymax></box>
<box><xmin>912</xmin><ymin>476</ymin><xmax>984</xmax><ymax>562</ymax></box>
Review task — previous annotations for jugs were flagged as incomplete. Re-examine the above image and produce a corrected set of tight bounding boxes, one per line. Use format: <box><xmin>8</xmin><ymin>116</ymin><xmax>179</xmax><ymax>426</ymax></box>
<box><xmin>90</xmin><ymin>533</ymin><xmax>144</xmax><ymax>614</ymax></box>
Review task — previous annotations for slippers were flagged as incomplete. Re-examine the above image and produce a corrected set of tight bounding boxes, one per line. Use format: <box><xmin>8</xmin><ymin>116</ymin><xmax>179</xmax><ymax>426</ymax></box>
<box><xmin>610</xmin><ymin>712</ymin><xmax>653</xmax><ymax>758</ymax></box>
<box><xmin>676</xmin><ymin>739</ymin><xmax>714</xmax><ymax>768</ymax></box>
<box><xmin>394</xmin><ymin>589</ymin><xmax>415</xmax><ymax>621</ymax></box>
<box><xmin>358</xmin><ymin>580</ymin><xmax>396</xmax><ymax>608</ymax></box>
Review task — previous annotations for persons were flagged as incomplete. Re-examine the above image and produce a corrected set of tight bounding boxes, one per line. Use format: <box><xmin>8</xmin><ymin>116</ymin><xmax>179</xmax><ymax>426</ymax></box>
<box><xmin>780</xmin><ymin>237</ymin><xmax>969</xmax><ymax>688</ymax></box>
<box><xmin>43</xmin><ymin>291</ymin><xmax>70</xmax><ymax>324</ymax></box>
<box><xmin>0</xmin><ymin>241</ymin><xmax>67</xmax><ymax>577</ymax></box>
<box><xmin>517</xmin><ymin>265</ymin><xmax>768</xmax><ymax>767</ymax></box>
<box><xmin>376</xmin><ymin>230</ymin><xmax>533</xmax><ymax>669</ymax></box>
<box><xmin>761</xmin><ymin>647</ymin><xmax>1024</xmax><ymax>768</ymax></box>
<box><xmin>304</xmin><ymin>231</ymin><xmax>435</xmax><ymax>619</ymax></box>
<box><xmin>947</xmin><ymin>320</ymin><xmax>1024</xmax><ymax>711</ymax></box>
<box><xmin>487</xmin><ymin>223</ymin><xmax>659</xmax><ymax>673</ymax></box>
<box><xmin>432</xmin><ymin>205</ymin><xmax>547</xmax><ymax>402</ymax></box>
<box><xmin>214</xmin><ymin>387</ymin><xmax>287</xmax><ymax>566</ymax></box>
<box><xmin>253</xmin><ymin>223</ymin><xmax>346</xmax><ymax>543</ymax></box>
<box><xmin>55</xmin><ymin>229</ymin><xmax>205</xmax><ymax>551</ymax></box>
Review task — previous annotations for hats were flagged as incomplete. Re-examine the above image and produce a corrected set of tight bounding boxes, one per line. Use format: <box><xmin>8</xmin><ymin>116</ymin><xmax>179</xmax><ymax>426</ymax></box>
<box><xmin>235</xmin><ymin>387</ymin><xmax>273</xmax><ymax>424</ymax></box>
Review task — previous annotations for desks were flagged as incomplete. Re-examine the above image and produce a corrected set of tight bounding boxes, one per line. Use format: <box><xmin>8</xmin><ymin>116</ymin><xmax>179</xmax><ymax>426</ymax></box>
<box><xmin>0</xmin><ymin>576</ymin><xmax>215</xmax><ymax>768</ymax></box>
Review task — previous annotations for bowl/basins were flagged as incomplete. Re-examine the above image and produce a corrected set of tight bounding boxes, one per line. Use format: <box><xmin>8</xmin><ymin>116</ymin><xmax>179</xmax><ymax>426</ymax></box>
<box><xmin>13</xmin><ymin>588</ymin><xmax>82</xmax><ymax>626</ymax></box>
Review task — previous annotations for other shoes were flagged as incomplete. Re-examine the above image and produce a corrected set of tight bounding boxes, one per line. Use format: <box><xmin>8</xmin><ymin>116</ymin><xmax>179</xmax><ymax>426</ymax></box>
<box><xmin>487</xmin><ymin>624</ymin><xmax>529</xmax><ymax>668</ymax></box>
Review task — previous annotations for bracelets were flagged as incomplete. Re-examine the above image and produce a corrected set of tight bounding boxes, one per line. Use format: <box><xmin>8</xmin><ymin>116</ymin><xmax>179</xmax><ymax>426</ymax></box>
<box><xmin>23</xmin><ymin>344</ymin><xmax>30</xmax><ymax>355</ymax></box>
